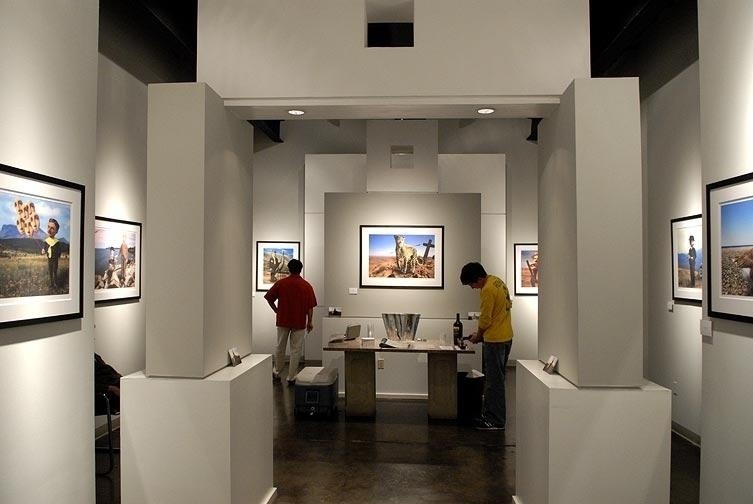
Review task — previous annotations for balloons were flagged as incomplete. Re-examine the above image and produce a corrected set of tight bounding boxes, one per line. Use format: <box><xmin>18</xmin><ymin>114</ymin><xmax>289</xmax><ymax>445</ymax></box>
<box><xmin>14</xmin><ymin>197</ymin><xmax>41</xmax><ymax>237</ymax></box>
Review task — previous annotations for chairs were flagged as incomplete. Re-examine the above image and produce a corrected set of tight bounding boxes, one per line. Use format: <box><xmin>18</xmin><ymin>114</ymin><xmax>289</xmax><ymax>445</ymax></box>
<box><xmin>95</xmin><ymin>392</ymin><xmax>120</xmax><ymax>477</ymax></box>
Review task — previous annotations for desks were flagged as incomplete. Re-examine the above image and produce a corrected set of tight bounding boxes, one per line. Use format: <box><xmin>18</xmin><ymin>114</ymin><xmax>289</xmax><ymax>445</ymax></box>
<box><xmin>323</xmin><ymin>335</ymin><xmax>475</xmax><ymax>419</ymax></box>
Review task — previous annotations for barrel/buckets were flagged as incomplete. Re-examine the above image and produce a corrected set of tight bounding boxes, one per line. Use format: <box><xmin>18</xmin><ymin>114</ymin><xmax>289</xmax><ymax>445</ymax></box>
<box><xmin>382</xmin><ymin>313</ymin><xmax>421</xmax><ymax>342</ymax></box>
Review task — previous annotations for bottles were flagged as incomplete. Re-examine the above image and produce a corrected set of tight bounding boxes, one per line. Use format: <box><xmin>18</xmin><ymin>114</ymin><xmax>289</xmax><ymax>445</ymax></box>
<box><xmin>462</xmin><ymin>335</ymin><xmax>484</xmax><ymax>343</ymax></box>
<box><xmin>453</xmin><ymin>313</ymin><xmax>463</xmax><ymax>345</ymax></box>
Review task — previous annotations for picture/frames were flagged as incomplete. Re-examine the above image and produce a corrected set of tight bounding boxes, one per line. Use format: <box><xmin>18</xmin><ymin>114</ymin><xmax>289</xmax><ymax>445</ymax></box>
<box><xmin>229</xmin><ymin>348</ymin><xmax>241</xmax><ymax>367</ymax></box>
<box><xmin>705</xmin><ymin>172</ymin><xmax>753</xmax><ymax>324</ymax></box>
<box><xmin>0</xmin><ymin>164</ymin><xmax>85</xmax><ymax>328</ymax></box>
<box><xmin>359</xmin><ymin>225</ymin><xmax>444</xmax><ymax>290</ymax></box>
<box><xmin>670</xmin><ymin>214</ymin><xmax>702</xmax><ymax>304</ymax></box>
<box><xmin>542</xmin><ymin>355</ymin><xmax>559</xmax><ymax>374</ymax></box>
<box><xmin>256</xmin><ymin>241</ymin><xmax>300</xmax><ymax>292</ymax></box>
<box><xmin>514</xmin><ymin>243</ymin><xmax>538</xmax><ymax>296</ymax></box>
<box><xmin>95</xmin><ymin>215</ymin><xmax>142</xmax><ymax>303</ymax></box>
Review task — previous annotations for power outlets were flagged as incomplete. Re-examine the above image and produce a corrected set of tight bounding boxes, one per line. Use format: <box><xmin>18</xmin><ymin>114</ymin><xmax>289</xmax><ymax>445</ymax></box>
<box><xmin>377</xmin><ymin>359</ymin><xmax>384</xmax><ymax>369</ymax></box>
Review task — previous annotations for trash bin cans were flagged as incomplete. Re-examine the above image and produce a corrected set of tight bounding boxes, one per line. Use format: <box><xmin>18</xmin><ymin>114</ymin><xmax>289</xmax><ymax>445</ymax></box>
<box><xmin>457</xmin><ymin>372</ymin><xmax>485</xmax><ymax>426</ymax></box>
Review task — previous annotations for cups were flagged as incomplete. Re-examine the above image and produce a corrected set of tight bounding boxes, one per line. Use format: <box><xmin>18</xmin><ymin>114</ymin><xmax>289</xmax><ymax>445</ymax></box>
<box><xmin>457</xmin><ymin>338</ymin><xmax>468</xmax><ymax>349</ymax></box>
<box><xmin>367</xmin><ymin>324</ymin><xmax>374</xmax><ymax>338</ymax></box>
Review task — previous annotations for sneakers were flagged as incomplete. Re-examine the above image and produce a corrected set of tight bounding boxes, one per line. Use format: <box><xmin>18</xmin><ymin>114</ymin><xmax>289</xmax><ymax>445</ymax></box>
<box><xmin>474</xmin><ymin>413</ymin><xmax>487</xmax><ymax>422</ymax></box>
<box><xmin>476</xmin><ymin>420</ymin><xmax>506</xmax><ymax>430</ymax></box>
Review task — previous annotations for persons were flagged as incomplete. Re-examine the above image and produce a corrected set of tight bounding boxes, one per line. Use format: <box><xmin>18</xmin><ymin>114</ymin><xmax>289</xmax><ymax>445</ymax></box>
<box><xmin>95</xmin><ymin>237</ymin><xmax>129</xmax><ymax>289</ymax></box>
<box><xmin>460</xmin><ymin>262</ymin><xmax>514</xmax><ymax>431</ymax></box>
<box><xmin>687</xmin><ymin>236</ymin><xmax>697</xmax><ymax>287</ymax></box>
<box><xmin>263</xmin><ymin>259</ymin><xmax>317</xmax><ymax>385</ymax></box>
<box><xmin>269</xmin><ymin>251</ymin><xmax>281</xmax><ymax>282</ymax></box>
<box><xmin>94</xmin><ymin>353</ymin><xmax>123</xmax><ymax>416</ymax></box>
<box><xmin>41</xmin><ymin>218</ymin><xmax>60</xmax><ymax>288</ymax></box>
